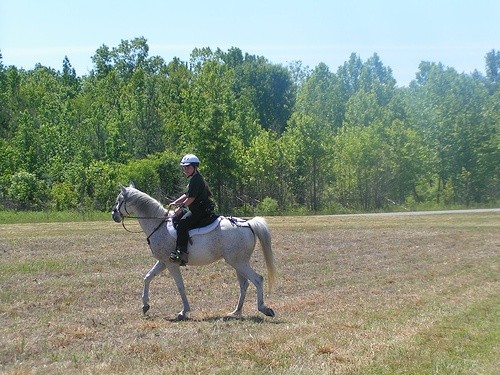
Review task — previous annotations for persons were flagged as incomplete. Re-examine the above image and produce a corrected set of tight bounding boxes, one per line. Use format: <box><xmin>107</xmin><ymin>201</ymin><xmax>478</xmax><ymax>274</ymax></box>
<box><xmin>166</xmin><ymin>153</ymin><xmax>219</xmax><ymax>267</ymax></box>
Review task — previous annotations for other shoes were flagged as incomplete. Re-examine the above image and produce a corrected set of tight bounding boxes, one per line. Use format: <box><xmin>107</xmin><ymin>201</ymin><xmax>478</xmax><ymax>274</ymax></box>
<box><xmin>171</xmin><ymin>251</ymin><xmax>189</xmax><ymax>262</ymax></box>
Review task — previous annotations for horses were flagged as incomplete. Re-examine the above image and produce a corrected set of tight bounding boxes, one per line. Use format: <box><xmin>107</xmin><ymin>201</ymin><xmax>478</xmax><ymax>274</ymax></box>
<box><xmin>112</xmin><ymin>180</ymin><xmax>276</xmax><ymax>321</ymax></box>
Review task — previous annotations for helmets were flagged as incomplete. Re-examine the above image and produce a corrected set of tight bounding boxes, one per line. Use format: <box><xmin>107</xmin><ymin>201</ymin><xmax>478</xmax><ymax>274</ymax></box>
<box><xmin>179</xmin><ymin>153</ymin><xmax>200</xmax><ymax>166</ymax></box>
